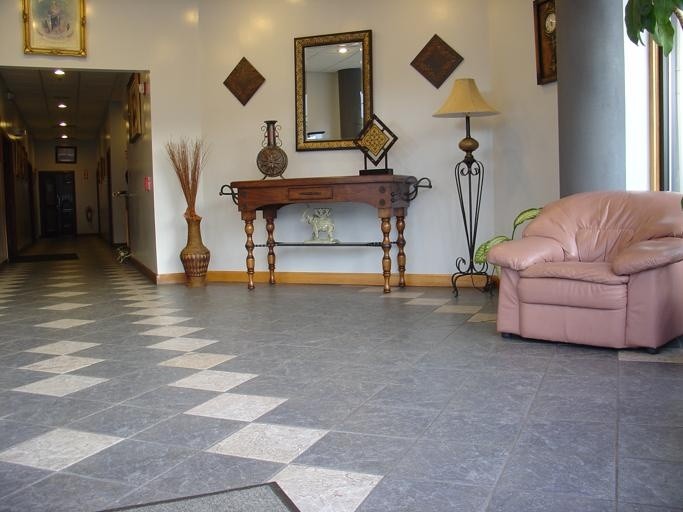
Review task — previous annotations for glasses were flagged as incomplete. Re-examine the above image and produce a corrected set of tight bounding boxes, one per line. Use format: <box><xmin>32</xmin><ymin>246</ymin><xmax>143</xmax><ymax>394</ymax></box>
<box><xmin>431</xmin><ymin>77</ymin><xmax>503</xmax><ymax>297</ymax></box>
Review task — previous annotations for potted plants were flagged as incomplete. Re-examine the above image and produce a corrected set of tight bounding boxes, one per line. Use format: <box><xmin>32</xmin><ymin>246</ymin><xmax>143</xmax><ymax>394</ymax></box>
<box><xmin>24</xmin><ymin>0</ymin><xmax>88</xmax><ymax>57</ymax></box>
<box><xmin>126</xmin><ymin>71</ymin><xmax>143</xmax><ymax>142</ymax></box>
<box><xmin>56</xmin><ymin>146</ymin><xmax>77</xmax><ymax>163</ymax></box>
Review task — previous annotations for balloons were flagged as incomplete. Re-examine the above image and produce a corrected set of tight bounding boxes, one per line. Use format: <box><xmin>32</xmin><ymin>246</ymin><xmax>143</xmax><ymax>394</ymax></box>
<box><xmin>542</xmin><ymin>0</ymin><xmax>557</xmax><ymax>73</ymax></box>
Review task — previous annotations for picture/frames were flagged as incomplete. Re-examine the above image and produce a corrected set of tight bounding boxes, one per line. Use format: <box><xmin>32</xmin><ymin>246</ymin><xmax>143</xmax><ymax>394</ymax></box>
<box><xmin>220</xmin><ymin>174</ymin><xmax>432</xmax><ymax>293</ymax></box>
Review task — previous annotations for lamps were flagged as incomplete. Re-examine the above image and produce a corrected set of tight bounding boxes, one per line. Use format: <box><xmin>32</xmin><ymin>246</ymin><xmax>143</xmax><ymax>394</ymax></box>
<box><xmin>485</xmin><ymin>189</ymin><xmax>683</xmax><ymax>356</ymax></box>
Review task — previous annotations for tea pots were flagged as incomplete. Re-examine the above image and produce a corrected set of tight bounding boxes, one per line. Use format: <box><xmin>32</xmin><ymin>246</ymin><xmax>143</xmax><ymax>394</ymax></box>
<box><xmin>293</xmin><ymin>29</ymin><xmax>373</xmax><ymax>152</ymax></box>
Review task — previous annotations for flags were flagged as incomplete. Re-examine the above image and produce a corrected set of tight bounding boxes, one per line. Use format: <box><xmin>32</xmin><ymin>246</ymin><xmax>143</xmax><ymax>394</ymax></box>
<box><xmin>180</xmin><ymin>218</ymin><xmax>211</xmax><ymax>288</ymax></box>
